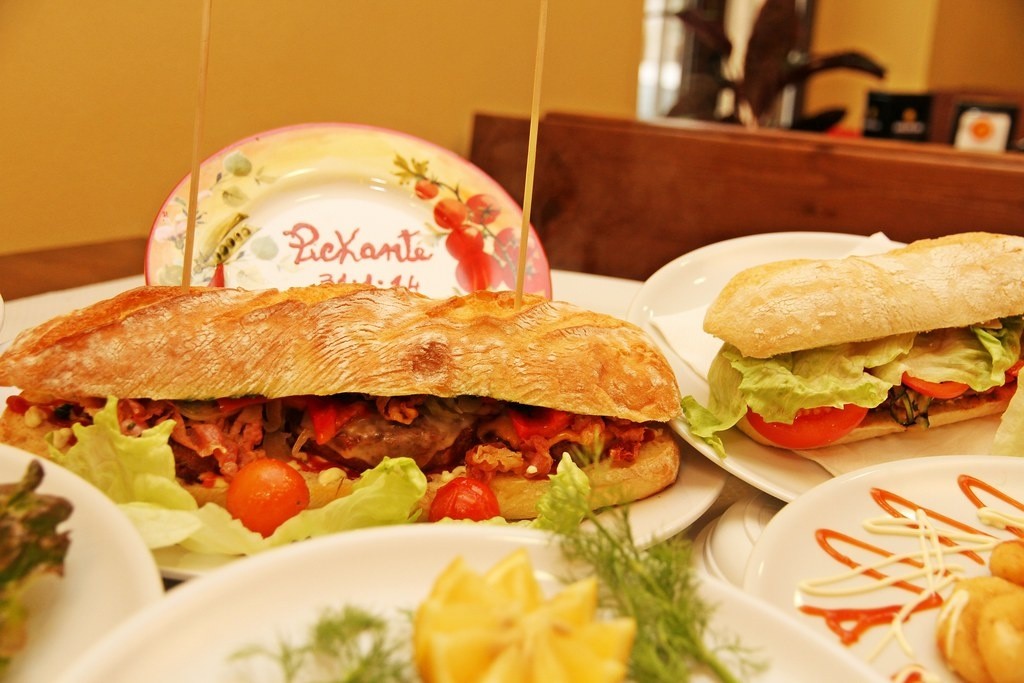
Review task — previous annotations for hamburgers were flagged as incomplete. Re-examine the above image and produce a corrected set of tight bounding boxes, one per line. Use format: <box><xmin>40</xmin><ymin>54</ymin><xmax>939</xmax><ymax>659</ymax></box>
<box><xmin>0</xmin><ymin>285</ymin><xmax>684</xmax><ymax>554</ymax></box>
<box><xmin>679</xmin><ymin>229</ymin><xmax>1023</xmax><ymax>461</ymax></box>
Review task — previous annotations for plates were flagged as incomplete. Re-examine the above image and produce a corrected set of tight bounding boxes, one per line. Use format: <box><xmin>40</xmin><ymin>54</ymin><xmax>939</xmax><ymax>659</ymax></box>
<box><xmin>0</xmin><ymin>442</ymin><xmax>165</xmax><ymax>683</ymax></box>
<box><xmin>627</xmin><ymin>229</ymin><xmax>1024</xmax><ymax>503</ymax></box>
<box><xmin>744</xmin><ymin>455</ymin><xmax>1024</xmax><ymax>683</ymax></box>
<box><xmin>0</xmin><ymin>272</ymin><xmax>728</xmax><ymax>582</ymax></box>
<box><xmin>143</xmin><ymin>121</ymin><xmax>553</xmax><ymax>304</ymax></box>
<box><xmin>66</xmin><ymin>522</ymin><xmax>883</xmax><ymax>683</ymax></box>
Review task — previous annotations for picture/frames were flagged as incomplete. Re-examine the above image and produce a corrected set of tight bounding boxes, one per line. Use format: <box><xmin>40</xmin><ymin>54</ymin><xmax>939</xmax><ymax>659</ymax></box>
<box><xmin>945</xmin><ymin>103</ymin><xmax>1018</xmax><ymax>153</ymax></box>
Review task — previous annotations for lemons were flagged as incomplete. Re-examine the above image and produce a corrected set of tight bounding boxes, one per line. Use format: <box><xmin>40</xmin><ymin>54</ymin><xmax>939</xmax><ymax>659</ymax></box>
<box><xmin>410</xmin><ymin>546</ymin><xmax>638</xmax><ymax>683</ymax></box>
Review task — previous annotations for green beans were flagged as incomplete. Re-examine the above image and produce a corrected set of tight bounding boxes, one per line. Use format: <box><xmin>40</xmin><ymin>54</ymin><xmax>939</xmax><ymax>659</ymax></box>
<box><xmin>221</xmin><ymin>429</ymin><xmax>780</xmax><ymax>683</ymax></box>
